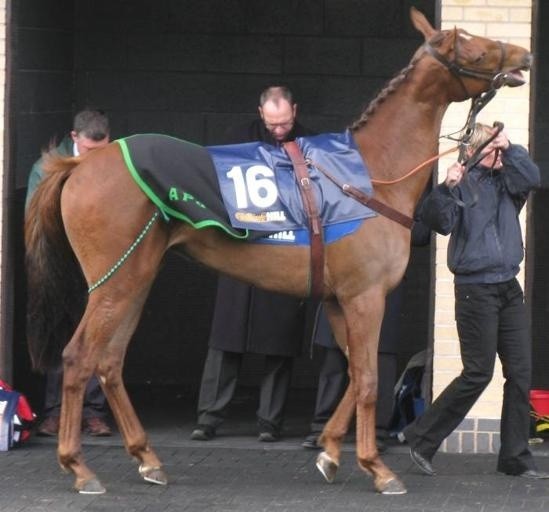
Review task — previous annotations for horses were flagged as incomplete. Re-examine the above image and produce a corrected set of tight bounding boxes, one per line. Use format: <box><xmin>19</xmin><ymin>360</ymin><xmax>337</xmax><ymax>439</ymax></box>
<box><xmin>22</xmin><ymin>5</ymin><xmax>534</xmax><ymax>495</ymax></box>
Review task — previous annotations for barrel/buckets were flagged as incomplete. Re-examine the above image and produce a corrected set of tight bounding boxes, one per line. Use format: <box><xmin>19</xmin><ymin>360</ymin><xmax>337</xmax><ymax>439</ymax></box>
<box><xmin>413</xmin><ymin>398</ymin><xmax>425</xmax><ymax>416</ymax></box>
<box><xmin>529</xmin><ymin>390</ymin><xmax>549</xmax><ymax>416</ymax></box>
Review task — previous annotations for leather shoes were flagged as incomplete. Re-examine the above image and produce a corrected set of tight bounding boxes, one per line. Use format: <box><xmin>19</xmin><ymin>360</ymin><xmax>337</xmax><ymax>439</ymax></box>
<box><xmin>398</xmin><ymin>430</ymin><xmax>435</xmax><ymax>475</ymax></box>
<box><xmin>498</xmin><ymin>468</ymin><xmax>549</xmax><ymax>479</ymax></box>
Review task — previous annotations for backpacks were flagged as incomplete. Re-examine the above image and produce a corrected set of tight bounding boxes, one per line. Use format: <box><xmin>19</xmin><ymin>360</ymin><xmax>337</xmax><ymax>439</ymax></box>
<box><xmin>391</xmin><ymin>365</ymin><xmax>426</xmax><ymax>430</ymax></box>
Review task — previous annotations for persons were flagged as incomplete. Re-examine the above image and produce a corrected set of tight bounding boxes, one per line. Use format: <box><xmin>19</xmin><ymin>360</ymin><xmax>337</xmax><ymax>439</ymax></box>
<box><xmin>187</xmin><ymin>84</ymin><xmax>321</xmax><ymax>441</ymax></box>
<box><xmin>23</xmin><ymin>110</ymin><xmax>115</xmax><ymax>437</ymax></box>
<box><xmin>303</xmin><ymin>262</ymin><xmax>410</xmax><ymax>452</ymax></box>
<box><xmin>396</xmin><ymin>123</ymin><xmax>549</xmax><ymax>481</ymax></box>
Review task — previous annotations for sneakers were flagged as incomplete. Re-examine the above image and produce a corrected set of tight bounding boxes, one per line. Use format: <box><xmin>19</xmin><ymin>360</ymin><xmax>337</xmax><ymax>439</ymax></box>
<box><xmin>190</xmin><ymin>424</ymin><xmax>215</xmax><ymax>441</ymax></box>
<box><xmin>376</xmin><ymin>437</ymin><xmax>387</xmax><ymax>453</ymax></box>
<box><xmin>302</xmin><ymin>433</ymin><xmax>320</xmax><ymax>448</ymax></box>
<box><xmin>39</xmin><ymin>415</ymin><xmax>60</xmax><ymax>435</ymax></box>
<box><xmin>257</xmin><ymin>425</ymin><xmax>276</xmax><ymax>441</ymax></box>
<box><xmin>82</xmin><ymin>416</ymin><xmax>112</xmax><ymax>436</ymax></box>
